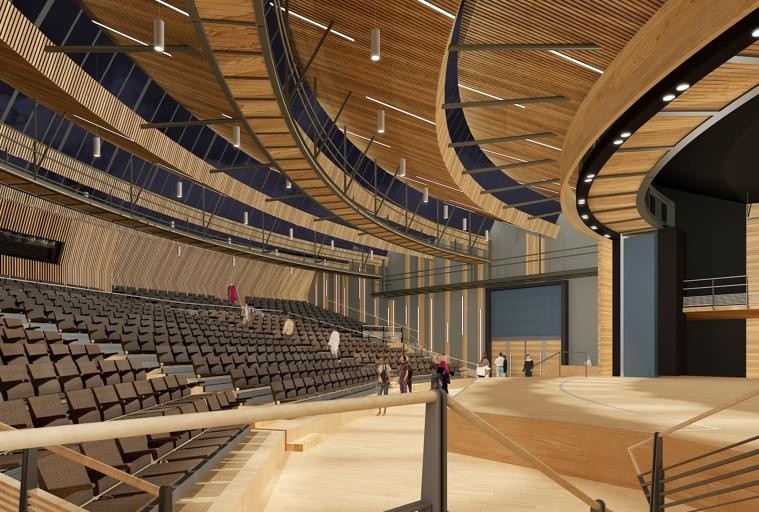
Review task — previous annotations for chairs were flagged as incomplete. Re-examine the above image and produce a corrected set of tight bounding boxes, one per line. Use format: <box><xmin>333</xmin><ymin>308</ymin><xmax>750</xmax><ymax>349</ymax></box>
<box><xmin>0</xmin><ymin>274</ymin><xmax>462</xmax><ymax>512</ymax></box>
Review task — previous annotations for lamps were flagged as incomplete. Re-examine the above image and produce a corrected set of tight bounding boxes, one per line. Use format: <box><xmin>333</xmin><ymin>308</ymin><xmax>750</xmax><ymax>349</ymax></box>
<box><xmin>378</xmin><ymin>109</ymin><xmax>384</xmax><ymax>134</ymax></box>
<box><xmin>154</xmin><ymin>1</ymin><xmax>165</xmax><ymax>52</ymax></box>
<box><xmin>399</xmin><ymin>158</ymin><xmax>405</xmax><ymax>177</ymax></box>
<box><xmin>244</xmin><ymin>212</ymin><xmax>248</xmax><ymax>225</ymax></box>
<box><xmin>370</xmin><ymin>0</ymin><xmax>381</xmax><ymax>61</ymax></box>
<box><xmin>422</xmin><ymin>186</ymin><xmax>428</xmax><ymax>202</ymax></box>
<box><xmin>232</xmin><ymin>125</ymin><xmax>241</xmax><ymax>147</ymax></box>
<box><xmin>289</xmin><ymin>228</ymin><xmax>374</xmax><ymax>261</ymax></box>
<box><xmin>443</xmin><ymin>205</ymin><xmax>488</xmax><ymax>242</ymax></box>
<box><xmin>176</xmin><ymin>181</ymin><xmax>182</xmax><ymax>197</ymax></box>
<box><xmin>92</xmin><ymin>136</ymin><xmax>100</xmax><ymax>156</ymax></box>
